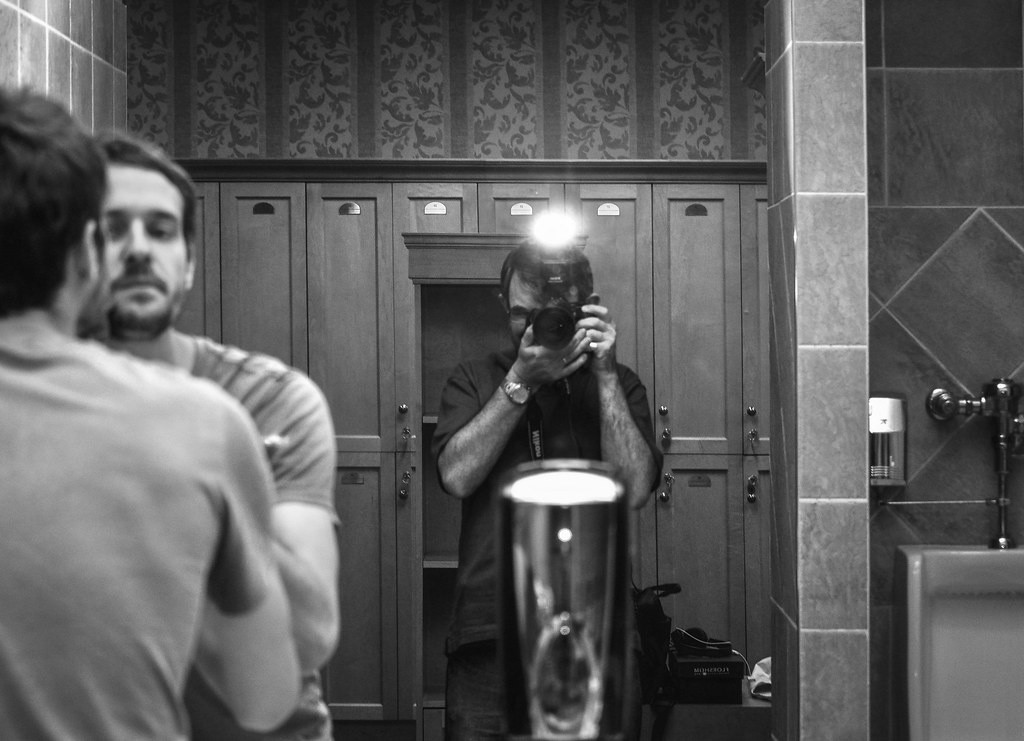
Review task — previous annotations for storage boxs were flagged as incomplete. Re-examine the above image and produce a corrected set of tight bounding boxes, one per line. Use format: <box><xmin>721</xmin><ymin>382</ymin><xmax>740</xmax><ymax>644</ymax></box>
<box><xmin>667</xmin><ymin>651</ymin><xmax>743</xmax><ymax>704</ymax></box>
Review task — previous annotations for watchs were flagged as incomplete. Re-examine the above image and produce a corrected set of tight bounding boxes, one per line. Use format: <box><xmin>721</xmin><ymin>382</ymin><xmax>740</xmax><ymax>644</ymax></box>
<box><xmin>500</xmin><ymin>378</ymin><xmax>536</xmax><ymax>405</ymax></box>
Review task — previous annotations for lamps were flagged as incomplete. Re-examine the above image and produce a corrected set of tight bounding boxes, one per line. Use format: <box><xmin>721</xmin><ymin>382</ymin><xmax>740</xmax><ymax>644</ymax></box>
<box><xmin>499</xmin><ymin>458</ymin><xmax>631</xmax><ymax>739</ymax></box>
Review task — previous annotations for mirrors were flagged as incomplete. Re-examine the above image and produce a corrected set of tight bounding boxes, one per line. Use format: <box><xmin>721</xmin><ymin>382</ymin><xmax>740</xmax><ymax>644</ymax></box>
<box><xmin>74</xmin><ymin>166</ymin><xmax>773</xmax><ymax>741</ymax></box>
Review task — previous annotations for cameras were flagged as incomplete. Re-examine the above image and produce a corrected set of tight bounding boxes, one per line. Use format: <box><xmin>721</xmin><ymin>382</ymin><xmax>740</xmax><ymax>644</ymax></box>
<box><xmin>524</xmin><ymin>210</ymin><xmax>599</xmax><ymax>351</ymax></box>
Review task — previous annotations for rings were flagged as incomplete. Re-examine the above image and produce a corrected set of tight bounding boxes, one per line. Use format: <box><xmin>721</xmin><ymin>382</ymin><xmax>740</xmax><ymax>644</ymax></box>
<box><xmin>589</xmin><ymin>341</ymin><xmax>598</xmax><ymax>352</ymax></box>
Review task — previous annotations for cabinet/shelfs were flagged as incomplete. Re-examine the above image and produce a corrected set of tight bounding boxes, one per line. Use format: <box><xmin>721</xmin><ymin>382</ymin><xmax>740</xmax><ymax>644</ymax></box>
<box><xmin>393</xmin><ymin>183</ymin><xmax>565</xmax><ymax>741</ymax></box>
<box><xmin>740</xmin><ymin>184</ymin><xmax>773</xmax><ymax>457</ymax></box>
<box><xmin>565</xmin><ymin>183</ymin><xmax>653</xmax><ymax>439</ymax></box>
<box><xmin>306</xmin><ymin>180</ymin><xmax>394</xmax><ymax>452</ymax></box>
<box><xmin>220</xmin><ymin>181</ymin><xmax>308</xmax><ymax>377</ymax></box>
<box><xmin>652</xmin><ymin>181</ymin><xmax>744</xmax><ymax>456</ymax></box>
<box><xmin>656</xmin><ymin>453</ymin><xmax>745</xmax><ymax>654</ymax></box>
<box><xmin>169</xmin><ymin>181</ymin><xmax>222</xmax><ymax>346</ymax></box>
<box><xmin>743</xmin><ymin>455</ymin><xmax>774</xmax><ymax>670</ymax></box>
<box><xmin>318</xmin><ymin>452</ymin><xmax>397</xmax><ymax>741</ymax></box>
<box><xmin>631</xmin><ymin>491</ymin><xmax>656</xmax><ymax>590</ymax></box>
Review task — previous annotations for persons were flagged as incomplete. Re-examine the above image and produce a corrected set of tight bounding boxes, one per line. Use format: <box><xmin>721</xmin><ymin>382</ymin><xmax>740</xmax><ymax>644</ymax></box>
<box><xmin>431</xmin><ymin>234</ymin><xmax>665</xmax><ymax>741</ymax></box>
<box><xmin>0</xmin><ymin>82</ymin><xmax>304</xmax><ymax>741</ymax></box>
<box><xmin>69</xmin><ymin>128</ymin><xmax>341</xmax><ymax>741</ymax></box>
